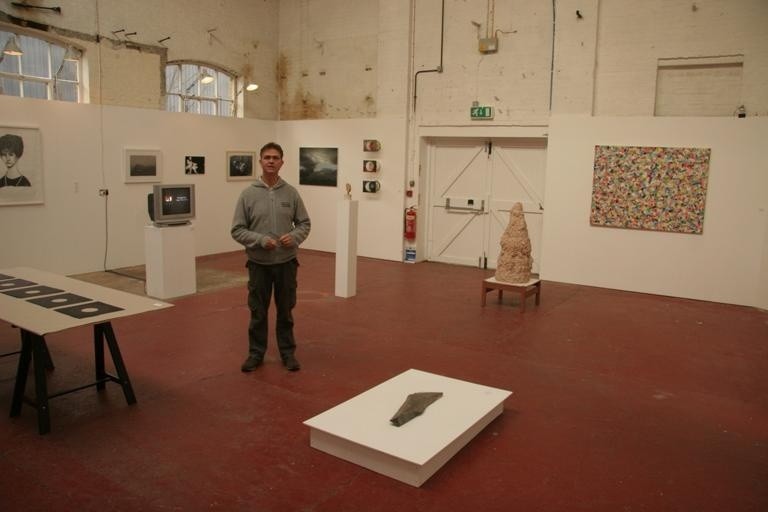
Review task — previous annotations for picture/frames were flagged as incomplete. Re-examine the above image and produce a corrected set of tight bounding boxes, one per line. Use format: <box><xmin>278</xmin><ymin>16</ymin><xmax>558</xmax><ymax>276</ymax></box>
<box><xmin>225</xmin><ymin>149</ymin><xmax>256</xmax><ymax>182</ymax></box>
<box><xmin>0</xmin><ymin>124</ymin><xmax>44</xmax><ymax>206</ymax></box>
<box><xmin>122</xmin><ymin>147</ymin><xmax>161</xmax><ymax>184</ymax></box>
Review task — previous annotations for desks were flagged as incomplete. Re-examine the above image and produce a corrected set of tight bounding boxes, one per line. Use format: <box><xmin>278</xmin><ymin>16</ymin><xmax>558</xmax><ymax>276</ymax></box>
<box><xmin>480</xmin><ymin>276</ymin><xmax>540</xmax><ymax>313</ymax></box>
<box><xmin>0</xmin><ymin>266</ymin><xmax>175</xmax><ymax>436</ymax></box>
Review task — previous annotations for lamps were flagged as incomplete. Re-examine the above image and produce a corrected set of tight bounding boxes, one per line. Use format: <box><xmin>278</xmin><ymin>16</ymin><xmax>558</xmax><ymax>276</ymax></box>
<box><xmin>55</xmin><ymin>45</ymin><xmax>80</xmax><ymax>75</ymax></box>
<box><xmin>2</xmin><ymin>36</ymin><xmax>23</xmax><ymax>56</ymax></box>
<box><xmin>186</xmin><ymin>69</ymin><xmax>213</xmax><ymax>91</ymax></box>
<box><xmin>236</xmin><ymin>80</ymin><xmax>258</xmax><ymax>99</ymax></box>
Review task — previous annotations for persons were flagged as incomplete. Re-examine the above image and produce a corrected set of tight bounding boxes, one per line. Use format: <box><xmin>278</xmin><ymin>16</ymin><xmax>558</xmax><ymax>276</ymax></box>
<box><xmin>230</xmin><ymin>142</ymin><xmax>313</xmax><ymax>373</ymax></box>
<box><xmin>0</xmin><ymin>134</ymin><xmax>31</xmax><ymax>190</ymax></box>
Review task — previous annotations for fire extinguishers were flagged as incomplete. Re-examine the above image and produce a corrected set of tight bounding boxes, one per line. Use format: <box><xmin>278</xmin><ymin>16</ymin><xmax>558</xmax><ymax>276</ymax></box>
<box><xmin>405</xmin><ymin>206</ymin><xmax>417</xmax><ymax>240</ymax></box>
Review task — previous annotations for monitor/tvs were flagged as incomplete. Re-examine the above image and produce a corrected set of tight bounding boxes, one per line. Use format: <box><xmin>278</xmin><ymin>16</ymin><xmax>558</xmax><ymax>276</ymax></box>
<box><xmin>147</xmin><ymin>184</ymin><xmax>196</xmax><ymax>228</ymax></box>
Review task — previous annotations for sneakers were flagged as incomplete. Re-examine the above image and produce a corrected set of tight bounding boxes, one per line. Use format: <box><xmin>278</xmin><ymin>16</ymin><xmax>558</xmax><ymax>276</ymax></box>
<box><xmin>241</xmin><ymin>358</ymin><xmax>263</xmax><ymax>371</ymax></box>
<box><xmin>283</xmin><ymin>357</ymin><xmax>300</xmax><ymax>371</ymax></box>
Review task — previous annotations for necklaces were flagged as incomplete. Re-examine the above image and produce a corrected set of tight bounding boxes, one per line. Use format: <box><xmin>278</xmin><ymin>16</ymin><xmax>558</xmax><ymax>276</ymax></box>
<box><xmin>3</xmin><ymin>171</ymin><xmax>23</xmax><ymax>187</ymax></box>
